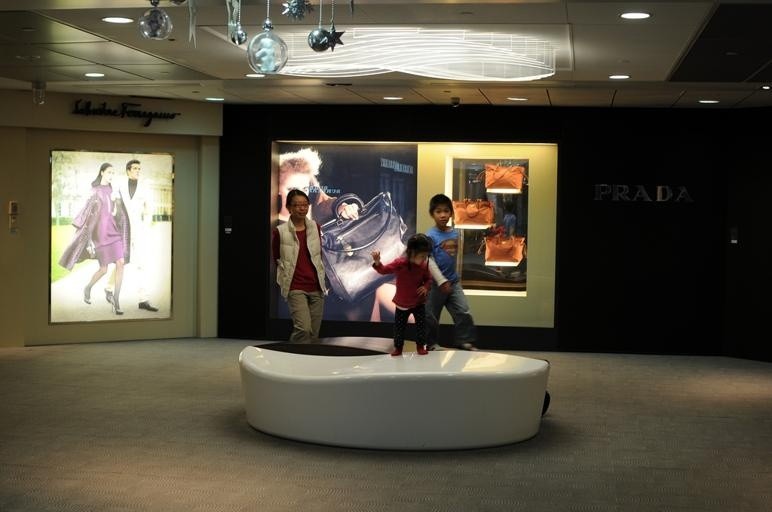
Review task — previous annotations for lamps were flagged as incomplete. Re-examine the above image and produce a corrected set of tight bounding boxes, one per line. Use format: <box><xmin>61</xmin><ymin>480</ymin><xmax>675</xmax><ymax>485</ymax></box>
<box><xmin>32</xmin><ymin>80</ymin><xmax>46</xmax><ymax>105</ymax></box>
<box><xmin>138</xmin><ymin>0</ymin><xmax>355</xmax><ymax>74</ymax></box>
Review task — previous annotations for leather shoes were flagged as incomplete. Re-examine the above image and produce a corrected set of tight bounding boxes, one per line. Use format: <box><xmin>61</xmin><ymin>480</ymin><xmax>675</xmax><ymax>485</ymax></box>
<box><xmin>83</xmin><ymin>286</ymin><xmax>159</xmax><ymax>314</ymax></box>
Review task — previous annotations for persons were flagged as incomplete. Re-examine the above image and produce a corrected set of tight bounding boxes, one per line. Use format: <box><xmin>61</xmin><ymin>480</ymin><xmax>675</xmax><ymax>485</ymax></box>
<box><xmin>278</xmin><ymin>148</ymin><xmax>417</xmax><ymax>324</ymax></box>
<box><xmin>271</xmin><ymin>189</ymin><xmax>328</xmax><ymax>343</ymax></box>
<box><xmin>104</xmin><ymin>159</ymin><xmax>159</xmax><ymax>312</ymax></box>
<box><xmin>371</xmin><ymin>233</ymin><xmax>433</xmax><ymax>356</ymax></box>
<box><xmin>502</xmin><ymin>200</ymin><xmax>517</xmax><ymax>237</ymax></box>
<box><xmin>422</xmin><ymin>194</ymin><xmax>479</xmax><ymax>351</ymax></box>
<box><xmin>58</xmin><ymin>163</ymin><xmax>131</xmax><ymax>314</ymax></box>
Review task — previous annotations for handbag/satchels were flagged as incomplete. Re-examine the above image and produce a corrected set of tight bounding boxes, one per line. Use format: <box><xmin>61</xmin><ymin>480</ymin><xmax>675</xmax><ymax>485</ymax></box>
<box><xmin>478</xmin><ymin>232</ymin><xmax>527</xmax><ymax>262</ymax></box>
<box><xmin>452</xmin><ymin>198</ymin><xmax>495</xmax><ymax>225</ymax></box>
<box><xmin>320</xmin><ymin>191</ymin><xmax>413</xmax><ymax>307</ymax></box>
<box><xmin>483</xmin><ymin>163</ymin><xmax>528</xmax><ymax>191</ymax></box>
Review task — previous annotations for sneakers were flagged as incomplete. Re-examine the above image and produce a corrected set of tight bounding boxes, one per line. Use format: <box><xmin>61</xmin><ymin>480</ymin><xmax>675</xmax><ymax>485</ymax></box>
<box><xmin>389</xmin><ymin>341</ymin><xmax>474</xmax><ymax>355</ymax></box>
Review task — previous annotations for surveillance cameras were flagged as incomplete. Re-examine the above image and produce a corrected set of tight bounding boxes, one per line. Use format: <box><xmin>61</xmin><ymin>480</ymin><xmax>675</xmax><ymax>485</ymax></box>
<box><xmin>449</xmin><ymin>97</ymin><xmax>460</xmax><ymax>108</ymax></box>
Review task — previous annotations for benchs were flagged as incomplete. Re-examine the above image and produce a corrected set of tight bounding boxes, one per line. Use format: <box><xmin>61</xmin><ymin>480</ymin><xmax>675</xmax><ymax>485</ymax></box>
<box><xmin>239</xmin><ymin>337</ymin><xmax>549</xmax><ymax>450</ymax></box>
